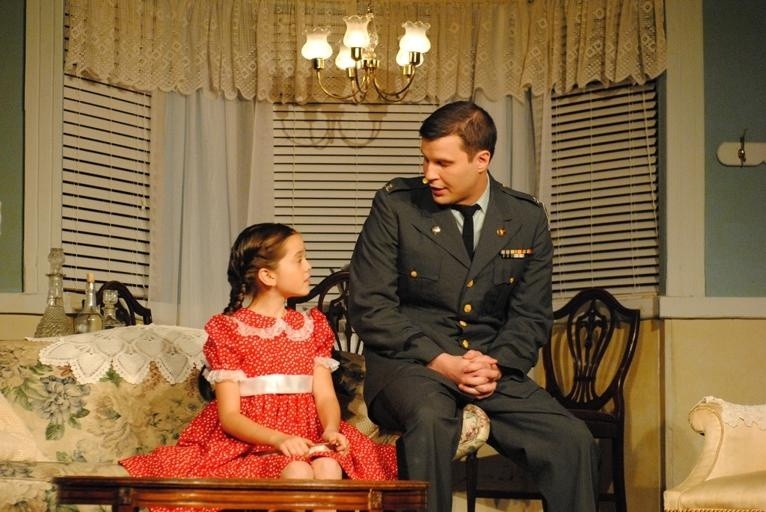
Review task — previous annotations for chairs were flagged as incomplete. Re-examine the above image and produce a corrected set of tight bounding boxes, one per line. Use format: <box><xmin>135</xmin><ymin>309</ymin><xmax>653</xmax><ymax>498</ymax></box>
<box><xmin>286</xmin><ymin>265</ymin><xmax>367</xmax><ymax>355</ymax></box>
<box><xmin>467</xmin><ymin>286</ymin><xmax>644</xmax><ymax>512</ymax></box>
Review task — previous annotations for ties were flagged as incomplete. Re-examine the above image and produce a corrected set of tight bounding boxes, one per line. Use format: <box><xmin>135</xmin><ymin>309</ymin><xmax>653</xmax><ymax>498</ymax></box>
<box><xmin>448</xmin><ymin>201</ymin><xmax>480</xmax><ymax>260</ymax></box>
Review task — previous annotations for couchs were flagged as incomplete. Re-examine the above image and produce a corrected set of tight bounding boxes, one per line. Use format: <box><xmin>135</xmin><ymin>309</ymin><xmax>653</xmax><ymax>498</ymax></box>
<box><xmin>0</xmin><ymin>323</ymin><xmax>490</xmax><ymax>512</ymax></box>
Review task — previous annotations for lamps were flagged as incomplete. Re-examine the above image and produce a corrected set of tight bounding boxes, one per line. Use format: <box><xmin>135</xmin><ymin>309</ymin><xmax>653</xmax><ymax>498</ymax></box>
<box><xmin>301</xmin><ymin>1</ymin><xmax>431</xmax><ymax>104</ymax></box>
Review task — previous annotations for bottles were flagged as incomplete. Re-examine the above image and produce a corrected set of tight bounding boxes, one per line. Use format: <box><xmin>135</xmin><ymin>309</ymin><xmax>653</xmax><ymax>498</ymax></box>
<box><xmin>100</xmin><ymin>289</ymin><xmax>124</xmax><ymax>330</ymax></box>
<box><xmin>35</xmin><ymin>247</ymin><xmax>73</xmax><ymax>338</ymax></box>
<box><xmin>73</xmin><ymin>272</ymin><xmax>104</xmax><ymax>334</ymax></box>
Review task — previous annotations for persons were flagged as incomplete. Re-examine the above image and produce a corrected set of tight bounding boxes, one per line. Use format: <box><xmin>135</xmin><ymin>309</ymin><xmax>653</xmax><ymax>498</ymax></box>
<box><xmin>118</xmin><ymin>222</ymin><xmax>398</xmax><ymax>512</ymax></box>
<box><xmin>349</xmin><ymin>101</ymin><xmax>602</xmax><ymax>512</ymax></box>
